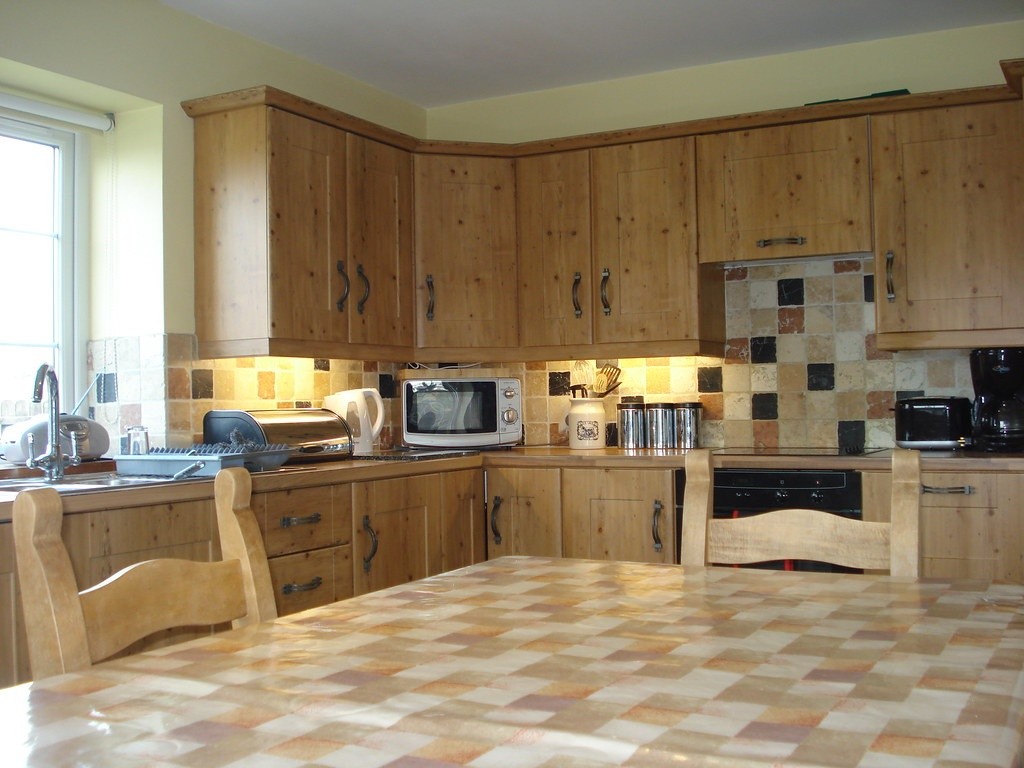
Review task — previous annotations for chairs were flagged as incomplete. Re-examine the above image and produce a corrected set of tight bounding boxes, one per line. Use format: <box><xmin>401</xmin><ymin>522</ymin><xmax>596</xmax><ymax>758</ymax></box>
<box><xmin>682</xmin><ymin>449</ymin><xmax>923</xmax><ymax>578</ymax></box>
<box><xmin>12</xmin><ymin>468</ymin><xmax>278</xmax><ymax>681</ymax></box>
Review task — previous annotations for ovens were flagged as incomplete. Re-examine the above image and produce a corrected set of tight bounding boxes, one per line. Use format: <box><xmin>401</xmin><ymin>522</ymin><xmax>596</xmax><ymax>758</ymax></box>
<box><xmin>672</xmin><ymin>466</ymin><xmax>864</xmax><ymax>575</ymax></box>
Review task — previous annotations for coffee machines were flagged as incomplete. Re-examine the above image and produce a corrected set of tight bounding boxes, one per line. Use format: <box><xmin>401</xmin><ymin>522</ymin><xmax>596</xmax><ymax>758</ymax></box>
<box><xmin>969</xmin><ymin>347</ymin><xmax>1024</xmax><ymax>455</ymax></box>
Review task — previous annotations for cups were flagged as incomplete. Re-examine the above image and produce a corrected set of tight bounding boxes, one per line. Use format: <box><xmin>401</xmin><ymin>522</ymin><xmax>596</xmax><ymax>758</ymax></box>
<box><xmin>839</xmin><ymin>419</ymin><xmax>866</xmax><ymax>455</ymax></box>
<box><xmin>126</xmin><ymin>425</ymin><xmax>149</xmax><ymax>455</ymax></box>
<box><xmin>606</xmin><ymin>422</ymin><xmax>618</xmax><ymax>446</ymax></box>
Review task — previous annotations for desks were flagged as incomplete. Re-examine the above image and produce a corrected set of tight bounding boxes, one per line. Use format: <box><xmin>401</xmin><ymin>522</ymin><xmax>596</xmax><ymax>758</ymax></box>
<box><xmin>0</xmin><ymin>555</ymin><xmax>1024</xmax><ymax>768</ymax></box>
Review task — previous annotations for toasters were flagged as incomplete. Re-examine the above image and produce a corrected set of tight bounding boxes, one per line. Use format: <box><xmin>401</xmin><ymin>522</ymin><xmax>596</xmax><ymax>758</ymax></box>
<box><xmin>894</xmin><ymin>396</ymin><xmax>973</xmax><ymax>449</ymax></box>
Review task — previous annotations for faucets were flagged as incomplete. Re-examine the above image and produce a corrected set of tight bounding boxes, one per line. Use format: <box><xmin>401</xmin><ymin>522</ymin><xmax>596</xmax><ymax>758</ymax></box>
<box><xmin>26</xmin><ymin>361</ymin><xmax>82</xmax><ymax>481</ymax></box>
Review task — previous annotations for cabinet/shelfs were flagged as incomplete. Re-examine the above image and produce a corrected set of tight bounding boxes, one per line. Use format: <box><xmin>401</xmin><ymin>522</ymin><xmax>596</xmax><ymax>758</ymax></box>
<box><xmin>857</xmin><ymin>470</ymin><xmax>1024</xmax><ymax>586</ymax></box>
<box><xmin>181</xmin><ymin>84</ymin><xmax>1024</xmax><ymax>361</ymax></box>
<box><xmin>0</xmin><ymin>466</ymin><xmax>674</xmax><ymax>689</ymax></box>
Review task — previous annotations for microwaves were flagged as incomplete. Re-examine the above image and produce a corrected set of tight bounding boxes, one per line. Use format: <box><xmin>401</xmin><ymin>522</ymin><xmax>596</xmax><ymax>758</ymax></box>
<box><xmin>400</xmin><ymin>377</ymin><xmax>522</xmax><ymax>454</ymax></box>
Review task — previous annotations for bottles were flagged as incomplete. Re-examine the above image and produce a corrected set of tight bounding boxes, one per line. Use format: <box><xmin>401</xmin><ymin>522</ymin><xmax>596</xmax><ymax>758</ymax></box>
<box><xmin>568</xmin><ymin>398</ymin><xmax>606</xmax><ymax>450</ymax></box>
<box><xmin>673</xmin><ymin>401</ymin><xmax>703</xmax><ymax>449</ymax></box>
<box><xmin>645</xmin><ymin>403</ymin><xmax>673</xmax><ymax>448</ymax></box>
<box><xmin>617</xmin><ymin>404</ymin><xmax>645</xmax><ymax>449</ymax></box>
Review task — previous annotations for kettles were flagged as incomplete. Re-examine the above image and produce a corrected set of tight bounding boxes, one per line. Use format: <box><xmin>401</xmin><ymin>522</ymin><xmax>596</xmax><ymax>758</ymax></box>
<box><xmin>323</xmin><ymin>387</ymin><xmax>385</xmax><ymax>458</ymax></box>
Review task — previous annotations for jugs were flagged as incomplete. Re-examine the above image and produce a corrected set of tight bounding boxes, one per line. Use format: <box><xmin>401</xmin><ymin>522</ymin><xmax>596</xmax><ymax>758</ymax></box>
<box><xmin>975</xmin><ymin>394</ymin><xmax>1023</xmax><ymax>433</ymax></box>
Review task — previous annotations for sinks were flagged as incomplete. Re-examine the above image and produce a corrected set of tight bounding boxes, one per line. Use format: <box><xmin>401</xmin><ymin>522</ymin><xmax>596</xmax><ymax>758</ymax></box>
<box><xmin>43</xmin><ymin>475</ymin><xmax>213</xmax><ymax>491</ymax></box>
<box><xmin>0</xmin><ymin>484</ymin><xmax>103</xmax><ymax>495</ymax></box>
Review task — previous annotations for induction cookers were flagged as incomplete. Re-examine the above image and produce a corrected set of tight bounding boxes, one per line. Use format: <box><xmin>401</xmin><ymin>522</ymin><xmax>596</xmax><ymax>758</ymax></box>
<box><xmin>712</xmin><ymin>446</ymin><xmax>842</xmax><ymax>456</ymax></box>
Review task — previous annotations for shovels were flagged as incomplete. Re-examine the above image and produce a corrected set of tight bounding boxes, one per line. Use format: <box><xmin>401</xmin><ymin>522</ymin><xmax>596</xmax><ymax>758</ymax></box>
<box><xmin>594</xmin><ymin>363</ymin><xmax>621</xmax><ymax>398</ymax></box>
